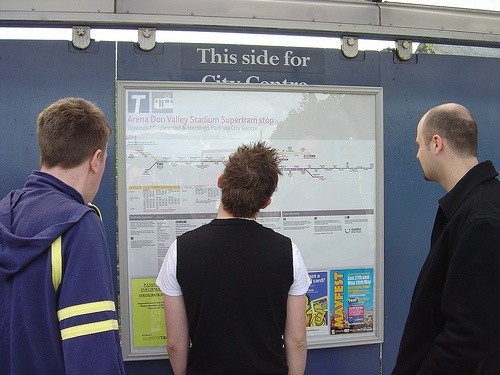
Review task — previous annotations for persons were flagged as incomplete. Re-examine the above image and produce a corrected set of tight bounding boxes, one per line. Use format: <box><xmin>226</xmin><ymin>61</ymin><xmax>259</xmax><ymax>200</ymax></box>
<box><xmin>154</xmin><ymin>140</ymin><xmax>312</xmax><ymax>375</ymax></box>
<box><xmin>391</xmin><ymin>102</ymin><xmax>500</xmax><ymax>375</ymax></box>
<box><xmin>0</xmin><ymin>96</ymin><xmax>126</xmax><ymax>375</ymax></box>
